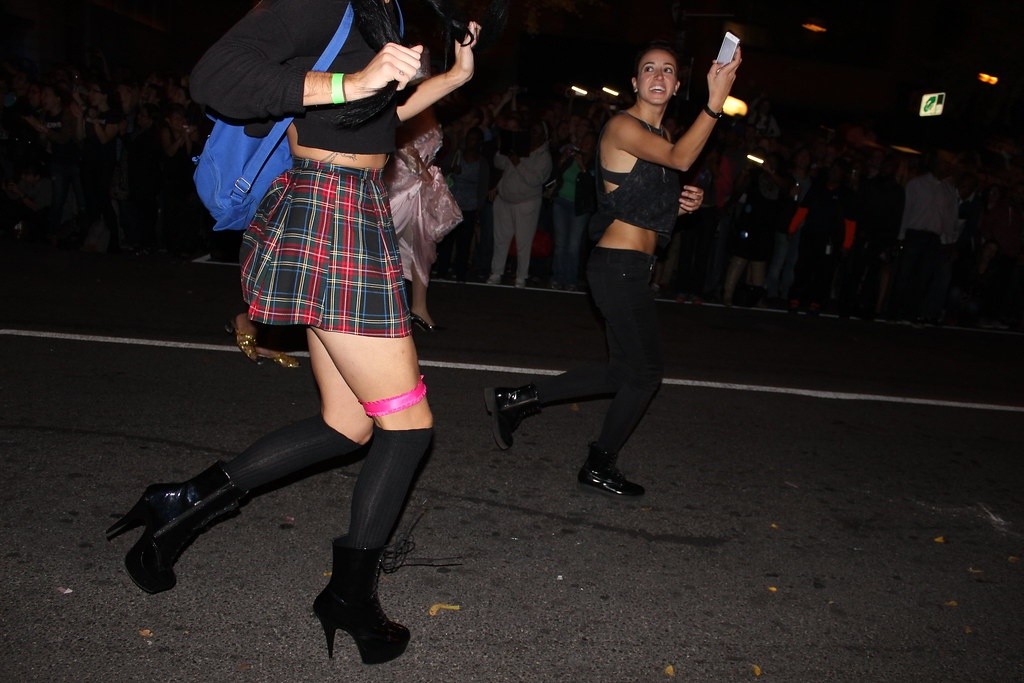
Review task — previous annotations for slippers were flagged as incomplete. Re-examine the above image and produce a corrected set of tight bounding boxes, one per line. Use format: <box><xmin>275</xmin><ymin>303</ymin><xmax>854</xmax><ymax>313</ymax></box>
<box><xmin>255</xmin><ymin>352</ymin><xmax>303</xmax><ymax>369</ymax></box>
<box><xmin>225</xmin><ymin>320</ymin><xmax>257</xmax><ymax>364</ymax></box>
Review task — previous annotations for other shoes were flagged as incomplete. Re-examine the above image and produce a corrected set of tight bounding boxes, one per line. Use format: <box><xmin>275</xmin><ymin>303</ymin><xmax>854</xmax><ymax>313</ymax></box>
<box><xmin>429</xmin><ymin>261</ymin><xmax>1023</xmax><ymax>327</ymax></box>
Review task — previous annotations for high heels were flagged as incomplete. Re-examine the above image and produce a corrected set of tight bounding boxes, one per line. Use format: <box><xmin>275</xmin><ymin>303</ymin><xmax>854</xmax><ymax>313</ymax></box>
<box><xmin>408</xmin><ymin>310</ymin><xmax>446</xmax><ymax>332</ymax></box>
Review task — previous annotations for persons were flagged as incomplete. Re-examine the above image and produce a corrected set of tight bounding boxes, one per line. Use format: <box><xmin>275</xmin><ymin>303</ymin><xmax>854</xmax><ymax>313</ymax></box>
<box><xmin>102</xmin><ymin>0</ymin><xmax>483</xmax><ymax>667</ymax></box>
<box><xmin>1</xmin><ymin>0</ymin><xmax>1023</xmax><ymax>372</ymax></box>
<box><xmin>482</xmin><ymin>44</ymin><xmax>742</xmax><ymax>502</ymax></box>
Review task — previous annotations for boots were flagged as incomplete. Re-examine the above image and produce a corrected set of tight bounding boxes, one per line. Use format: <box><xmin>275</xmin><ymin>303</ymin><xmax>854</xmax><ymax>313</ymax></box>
<box><xmin>313</xmin><ymin>538</ymin><xmax>410</xmax><ymax>664</ymax></box>
<box><xmin>577</xmin><ymin>442</ymin><xmax>646</xmax><ymax>502</ymax></box>
<box><xmin>106</xmin><ymin>460</ymin><xmax>246</xmax><ymax>594</ymax></box>
<box><xmin>484</xmin><ymin>384</ymin><xmax>542</xmax><ymax>449</ymax></box>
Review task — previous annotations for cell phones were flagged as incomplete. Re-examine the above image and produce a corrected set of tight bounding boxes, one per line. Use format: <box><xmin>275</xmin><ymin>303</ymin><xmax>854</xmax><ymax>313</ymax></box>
<box><xmin>715</xmin><ymin>31</ymin><xmax>740</xmax><ymax>75</ymax></box>
<box><xmin>570</xmin><ymin>144</ymin><xmax>580</xmax><ymax>159</ymax></box>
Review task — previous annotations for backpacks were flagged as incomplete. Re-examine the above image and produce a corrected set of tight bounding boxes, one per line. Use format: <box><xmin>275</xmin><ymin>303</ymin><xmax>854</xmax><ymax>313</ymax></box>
<box><xmin>192</xmin><ymin>0</ymin><xmax>404</xmax><ymax>231</ymax></box>
